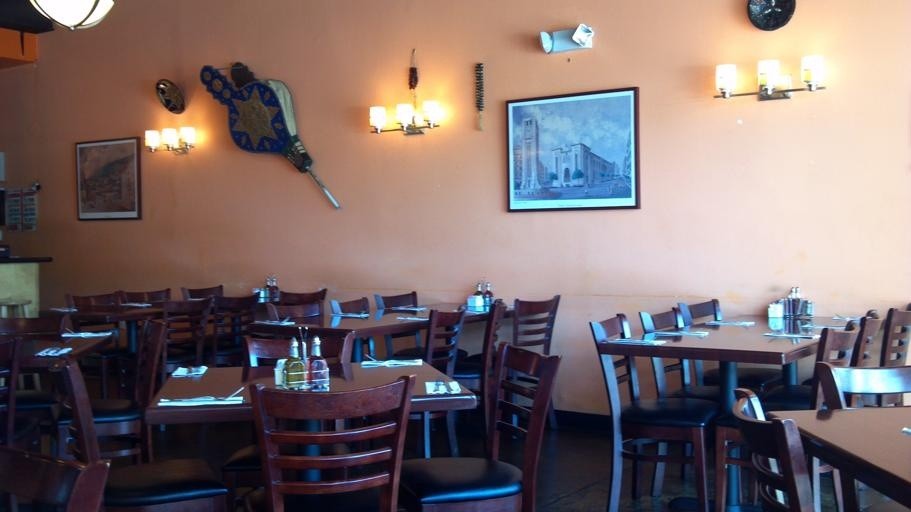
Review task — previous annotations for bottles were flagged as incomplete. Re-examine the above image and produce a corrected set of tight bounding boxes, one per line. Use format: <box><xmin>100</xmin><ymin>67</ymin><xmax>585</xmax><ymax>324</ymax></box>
<box><xmin>283</xmin><ymin>336</ymin><xmax>330</xmax><ymax>392</ymax></box>
<box><xmin>787</xmin><ymin>286</ymin><xmax>800</xmax><ymax>316</ymax></box>
<box><xmin>264</xmin><ymin>277</ymin><xmax>279</xmax><ymax>303</ymax></box>
<box><xmin>473</xmin><ymin>282</ymin><xmax>494</xmax><ymax>306</ymax></box>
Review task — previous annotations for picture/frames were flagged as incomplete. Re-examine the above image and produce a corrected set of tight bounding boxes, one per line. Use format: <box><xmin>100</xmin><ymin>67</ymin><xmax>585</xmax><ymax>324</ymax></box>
<box><xmin>75</xmin><ymin>136</ymin><xmax>143</xmax><ymax>221</ymax></box>
<box><xmin>506</xmin><ymin>86</ymin><xmax>641</xmax><ymax>213</ymax></box>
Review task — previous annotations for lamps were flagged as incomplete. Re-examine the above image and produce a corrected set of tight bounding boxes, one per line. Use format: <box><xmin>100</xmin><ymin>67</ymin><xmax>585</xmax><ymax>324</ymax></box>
<box><xmin>370</xmin><ymin>101</ymin><xmax>443</xmax><ymax>137</ymax></box>
<box><xmin>144</xmin><ymin>127</ymin><xmax>196</xmax><ymax>156</ymax></box>
<box><xmin>715</xmin><ymin>56</ymin><xmax>826</xmax><ymax>103</ymax></box>
